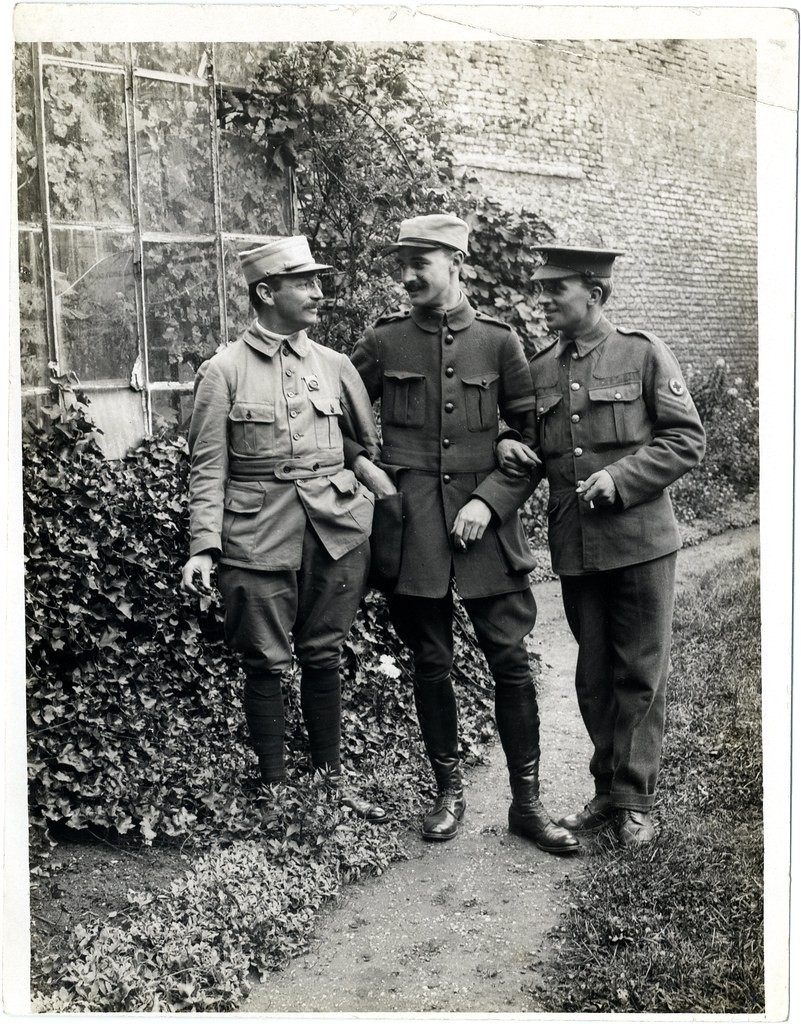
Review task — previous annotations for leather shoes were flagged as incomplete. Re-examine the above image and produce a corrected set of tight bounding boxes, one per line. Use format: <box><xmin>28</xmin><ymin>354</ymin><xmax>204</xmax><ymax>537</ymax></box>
<box><xmin>558</xmin><ymin>797</ymin><xmax>619</xmax><ymax>832</ymax></box>
<box><xmin>326</xmin><ymin>789</ymin><xmax>387</xmax><ymax>822</ymax></box>
<box><xmin>619</xmin><ymin>810</ymin><xmax>656</xmax><ymax>852</ymax></box>
<box><xmin>261</xmin><ymin>783</ymin><xmax>293</xmax><ymax>820</ymax></box>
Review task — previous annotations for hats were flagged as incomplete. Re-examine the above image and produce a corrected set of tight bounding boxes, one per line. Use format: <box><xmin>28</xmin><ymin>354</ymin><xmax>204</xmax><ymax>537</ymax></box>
<box><xmin>239</xmin><ymin>235</ymin><xmax>334</xmax><ymax>285</ymax></box>
<box><xmin>383</xmin><ymin>214</ymin><xmax>469</xmax><ymax>257</ymax></box>
<box><xmin>529</xmin><ymin>245</ymin><xmax>625</xmax><ymax>280</ymax></box>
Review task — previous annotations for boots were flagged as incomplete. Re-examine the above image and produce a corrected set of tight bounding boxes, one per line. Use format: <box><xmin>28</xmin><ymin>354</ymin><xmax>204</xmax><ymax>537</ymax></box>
<box><xmin>493</xmin><ymin>684</ymin><xmax>581</xmax><ymax>851</ymax></box>
<box><xmin>415</xmin><ymin>674</ymin><xmax>467</xmax><ymax>839</ymax></box>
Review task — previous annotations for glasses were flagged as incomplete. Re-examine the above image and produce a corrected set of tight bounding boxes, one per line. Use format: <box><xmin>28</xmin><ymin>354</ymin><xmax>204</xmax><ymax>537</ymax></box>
<box><xmin>281</xmin><ymin>279</ymin><xmax>322</xmax><ymax>291</ymax></box>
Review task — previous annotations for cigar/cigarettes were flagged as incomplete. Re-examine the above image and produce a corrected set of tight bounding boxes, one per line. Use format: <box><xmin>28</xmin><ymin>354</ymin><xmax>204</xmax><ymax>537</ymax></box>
<box><xmin>197</xmin><ymin>585</ymin><xmax>212</xmax><ymax>596</ymax></box>
<box><xmin>587</xmin><ymin>489</ymin><xmax>595</xmax><ymax>508</ymax></box>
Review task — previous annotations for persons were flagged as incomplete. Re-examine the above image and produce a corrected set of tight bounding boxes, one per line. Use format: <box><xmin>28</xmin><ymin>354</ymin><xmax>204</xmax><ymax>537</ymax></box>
<box><xmin>179</xmin><ymin>234</ymin><xmax>391</xmax><ymax>827</ymax></box>
<box><xmin>491</xmin><ymin>244</ymin><xmax>706</xmax><ymax>851</ymax></box>
<box><xmin>340</xmin><ymin>213</ymin><xmax>582</xmax><ymax>852</ymax></box>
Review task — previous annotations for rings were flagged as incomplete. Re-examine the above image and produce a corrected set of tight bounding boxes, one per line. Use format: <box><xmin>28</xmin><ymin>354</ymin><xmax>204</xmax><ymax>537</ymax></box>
<box><xmin>460</xmin><ymin>539</ymin><xmax>466</xmax><ymax>548</ymax></box>
